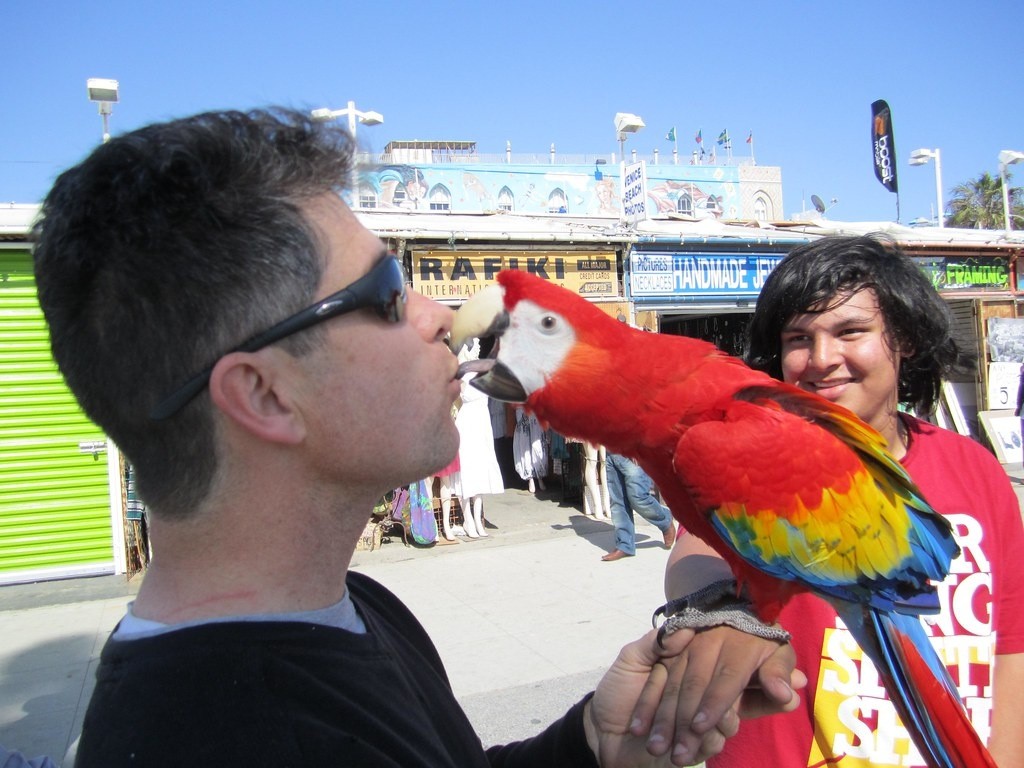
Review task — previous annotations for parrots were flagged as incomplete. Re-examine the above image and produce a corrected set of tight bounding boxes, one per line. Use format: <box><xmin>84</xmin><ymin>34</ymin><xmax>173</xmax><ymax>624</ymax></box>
<box><xmin>451</xmin><ymin>270</ymin><xmax>999</xmax><ymax>768</ymax></box>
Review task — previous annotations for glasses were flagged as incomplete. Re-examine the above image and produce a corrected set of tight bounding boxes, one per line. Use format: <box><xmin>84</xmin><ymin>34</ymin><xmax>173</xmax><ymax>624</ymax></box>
<box><xmin>150</xmin><ymin>255</ymin><xmax>407</xmax><ymax>420</ymax></box>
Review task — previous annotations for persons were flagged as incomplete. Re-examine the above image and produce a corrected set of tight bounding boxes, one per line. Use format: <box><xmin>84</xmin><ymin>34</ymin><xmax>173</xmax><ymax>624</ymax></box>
<box><xmin>602</xmin><ymin>449</ymin><xmax>676</xmax><ymax>561</ymax></box>
<box><xmin>630</xmin><ymin>234</ymin><xmax>1023</xmax><ymax>768</ymax></box>
<box><xmin>512</xmin><ymin>408</ymin><xmax>549</xmax><ymax>493</ymax></box>
<box><xmin>425</xmin><ymin>453</ymin><xmax>461</xmax><ymax>542</ymax></box>
<box><xmin>451</xmin><ymin>339</ymin><xmax>504</xmax><ymax>538</ymax></box>
<box><xmin>565</xmin><ymin>437</ymin><xmax>611</xmax><ymax>519</ymax></box>
<box><xmin>30</xmin><ymin>104</ymin><xmax>808</xmax><ymax>768</ymax></box>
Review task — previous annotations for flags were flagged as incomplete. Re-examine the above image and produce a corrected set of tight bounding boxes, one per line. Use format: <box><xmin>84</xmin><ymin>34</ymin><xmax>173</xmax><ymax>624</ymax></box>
<box><xmin>709</xmin><ymin>153</ymin><xmax>714</xmax><ymax>162</ymax></box>
<box><xmin>665</xmin><ymin>127</ymin><xmax>675</xmax><ymax>143</ymax></box>
<box><xmin>724</xmin><ymin>138</ymin><xmax>731</xmax><ymax>149</ymax></box>
<box><xmin>717</xmin><ymin>129</ymin><xmax>727</xmax><ymax>145</ymax></box>
<box><xmin>695</xmin><ymin>130</ymin><xmax>702</xmax><ymax>143</ymax></box>
<box><xmin>746</xmin><ymin>133</ymin><xmax>752</xmax><ymax>144</ymax></box>
<box><xmin>700</xmin><ymin>147</ymin><xmax>705</xmax><ymax>159</ymax></box>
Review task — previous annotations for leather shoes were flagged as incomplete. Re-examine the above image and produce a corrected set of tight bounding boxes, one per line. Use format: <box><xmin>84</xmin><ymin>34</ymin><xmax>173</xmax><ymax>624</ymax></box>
<box><xmin>663</xmin><ymin>514</ymin><xmax>676</xmax><ymax>548</ymax></box>
<box><xmin>602</xmin><ymin>549</ymin><xmax>630</xmax><ymax>561</ymax></box>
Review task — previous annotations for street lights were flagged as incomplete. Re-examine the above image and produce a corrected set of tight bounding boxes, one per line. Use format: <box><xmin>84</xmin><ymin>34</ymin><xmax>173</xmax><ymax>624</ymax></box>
<box><xmin>614</xmin><ymin>111</ymin><xmax>646</xmax><ymax>227</ymax></box>
<box><xmin>997</xmin><ymin>149</ymin><xmax>1024</xmax><ymax>230</ymax></box>
<box><xmin>86</xmin><ymin>76</ymin><xmax>130</xmax><ymax>577</ymax></box>
<box><xmin>908</xmin><ymin>147</ymin><xmax>944</xmax><ymax>228</ymax></box>
<box><xmin>308</xmin><ymin>99</ymin><xmax>385</xmax><ymax>221</ymax></box>
